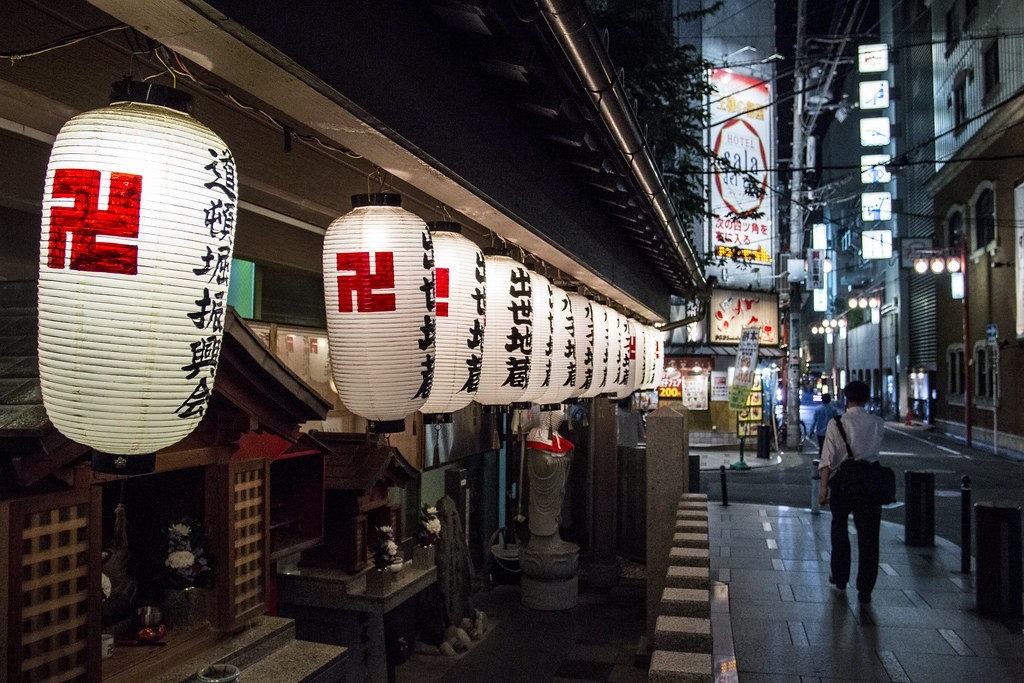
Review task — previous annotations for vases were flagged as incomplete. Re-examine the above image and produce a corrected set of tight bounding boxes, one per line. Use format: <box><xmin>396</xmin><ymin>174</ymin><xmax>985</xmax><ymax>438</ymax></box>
<box><xmin>101</xmin><ymin>632</ymin><xmax>117</xmax><ymax>661</ymax></box>
<box><xmin>165</xmin><ymin>585</ymin><xmax>212</xmax><ymax>631</ymax></box>
<box><xmin>384</xmin><ymin>557</ymin><xmax>404</xmax><ymax>573</ymax></box>
<box><xmin>412</xmin><ymin>544</ymin><xmax>436</xmax><ymax>571</ymax></box>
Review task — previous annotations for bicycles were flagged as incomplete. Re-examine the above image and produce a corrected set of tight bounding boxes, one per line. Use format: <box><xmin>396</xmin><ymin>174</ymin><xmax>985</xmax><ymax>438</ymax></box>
<box><xmin>778</xmin><ymin>411</ymin><xmax>807</xmax><ymax>445</ymax></box>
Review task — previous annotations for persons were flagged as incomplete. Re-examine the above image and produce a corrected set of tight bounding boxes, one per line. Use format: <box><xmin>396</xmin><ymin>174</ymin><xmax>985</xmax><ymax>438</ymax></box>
<box><xmin>808</xmin><ymin>391</ymin><xmax>839</xmax><ymax>461</ymax></box>
<box><xmin>816</xmin><ymin>381</ymin><xmax>886</xmax><ymax>603</ymax></box>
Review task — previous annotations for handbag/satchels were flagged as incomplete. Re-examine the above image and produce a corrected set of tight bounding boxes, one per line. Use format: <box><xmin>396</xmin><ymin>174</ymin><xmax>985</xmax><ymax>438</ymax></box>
<box><xmin>834</xmin><ymin>457</ymin><xmax>897</xmax><ymax>505</ymax></box>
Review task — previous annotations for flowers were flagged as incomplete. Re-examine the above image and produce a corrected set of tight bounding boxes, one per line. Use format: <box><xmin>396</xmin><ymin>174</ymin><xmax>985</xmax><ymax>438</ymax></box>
<box><xmin>414</xmin><ymin>503</ymin><xmax>442</xmax><ymax>549</ymax></box>
<box><xmin>370</xmin><ymin>525</ymin><xmax>399</xmax><ymax>572</ymax></box>
<box><xmin>165</xmin><ymin>521</ymin><xmax>212</xmax><ymax>613</ymax></box>
<box><xmin>100</xmin><ymin>571</ymin><xmax>112</xmax><ymax>603</ymax></box>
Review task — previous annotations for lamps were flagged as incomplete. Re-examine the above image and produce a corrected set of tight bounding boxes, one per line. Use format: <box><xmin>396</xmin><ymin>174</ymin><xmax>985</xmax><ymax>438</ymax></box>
<box><xmin>319</xmin><ymin>164</ymin><xmax>439</xmax><ymax>471</ymax></box>
<box><xmin>527</xmin><ymin>254</ymin><xmax>666</xmax><ymax>440</ymax></box>
<box><xmin>35</xmin><ymin>49</ymin><xmax>239</xmax><ymax>554</ymax></box>
<box><xmin>426</xmin><ymin>203</ymin><xmax>488</xmax><ymax>445</ymax></box>
<box><xmin>482</xmin><ymin>228</ymin><xmax>535</xmax><ymax>451</ymax></box>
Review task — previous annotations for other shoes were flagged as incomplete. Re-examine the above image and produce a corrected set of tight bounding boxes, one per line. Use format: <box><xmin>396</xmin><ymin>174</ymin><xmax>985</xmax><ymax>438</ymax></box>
<box><xmin>830</xmin><ymin>576</ymin><xmax>847</xmax><ymax>589</ymax></box>
<box><xmin>858</xmin><ymin>591</ymin><xmax>871</xmax><ymax>603</ymax></box>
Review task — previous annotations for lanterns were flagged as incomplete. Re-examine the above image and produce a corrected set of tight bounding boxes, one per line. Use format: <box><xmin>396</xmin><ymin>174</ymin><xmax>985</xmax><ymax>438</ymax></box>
<box><xmin>556</xmin><ymin>283</ymin><xmax>592</xmax><ymax>399</ymax></box>
<box><xmin>416</xmin><ymin>219</ymin><xmax>487</xmax><ymax>417</ymax></box>
<box><xmin>320</xmin><ymin>193</ymin><xmax>437</xmax><ymax>421</ymax></box>
<box><xmin>515</xmin><ymin>260</ymin><xmax>554</xmax><ymax>405</ymax></box>
<box><xmin>580</xmin><ymin>293</ymin><xmax>608</xmax><ymax>399</ymax></box>
<box><xmin>601</xmin><ymin>301</ymin><xmax>665</xmax><ymax>403</ymax></box>
<box><xmin>534</xmin><ymin>276</ymin><xmax>576</xmax><ymax>409</ymax></box>
<box><xmin>34</xmin><ymin>82</ymin><xmax>241</xmax><ymax>463</ymax></box>
<box><xmin>473</xmin><ymin>245</ymin><xmax>537</xmax><ymax>408</ymax></box>
